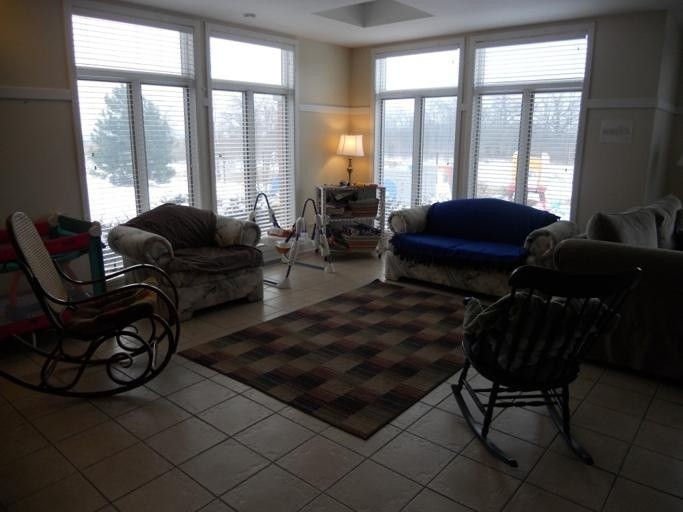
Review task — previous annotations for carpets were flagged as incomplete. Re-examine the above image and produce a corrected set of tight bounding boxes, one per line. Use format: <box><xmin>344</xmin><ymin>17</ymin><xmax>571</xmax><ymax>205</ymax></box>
<box><xmin>179</xmin><ymin>278</ymin><xmax>498</xmax><ymax>440</ymax></box>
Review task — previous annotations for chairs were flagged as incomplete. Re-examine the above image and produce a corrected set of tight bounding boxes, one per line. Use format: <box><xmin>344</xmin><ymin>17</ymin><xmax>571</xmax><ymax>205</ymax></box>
<box><xmin>0</xmin><ymin>208</ymin><xmax>180</xmax><ymax>400</ymax></box>
<box><xmin>451</xmin><ymin>263</ymin><xmax>643</xmax><ymax>468</ymax></box>
<box><xmin>108</xmin><ymin>203</ymin><xmax>265</xmax><ymax>324</ymax></box>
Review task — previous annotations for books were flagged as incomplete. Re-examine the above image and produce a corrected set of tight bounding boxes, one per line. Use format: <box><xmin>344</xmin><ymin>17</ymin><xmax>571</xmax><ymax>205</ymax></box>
<box><xmin>325</xmin><ymin>197</ymin><xmax>378</xmax><ymax>219</ymax></box>
<box><xmin>335</xmin><ymin>223</ymin><xmax>382</xmax><ymax>254</ymax></box>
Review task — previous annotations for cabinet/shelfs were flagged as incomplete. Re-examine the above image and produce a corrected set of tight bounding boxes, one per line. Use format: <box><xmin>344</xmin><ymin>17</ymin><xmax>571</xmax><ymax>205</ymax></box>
<box><xmin>313</xmin><ymin>183</ymin><xmax>385</xmax><ymax>262</ymax></box>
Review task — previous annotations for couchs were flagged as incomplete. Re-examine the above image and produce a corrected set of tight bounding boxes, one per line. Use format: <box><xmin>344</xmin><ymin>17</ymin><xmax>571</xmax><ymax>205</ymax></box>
<box><xmin>554</xmin><ymin>196</ymin><xmax>683</xmax><ymax>388</ymax></box>
<box><xmin>383</xmin><ymin>198</ymin><xmax>577</xmax><ymax>299</ymax></box>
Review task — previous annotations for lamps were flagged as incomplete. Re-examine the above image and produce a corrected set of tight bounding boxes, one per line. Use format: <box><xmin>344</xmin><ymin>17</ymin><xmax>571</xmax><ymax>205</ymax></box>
<box><xmin>334</xmin><ymin>132</ymin><xmax>366</xmax><ymax>187</ymax></box>
